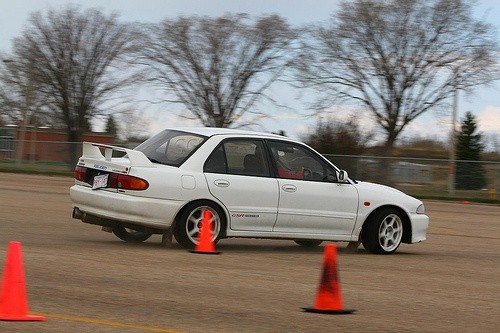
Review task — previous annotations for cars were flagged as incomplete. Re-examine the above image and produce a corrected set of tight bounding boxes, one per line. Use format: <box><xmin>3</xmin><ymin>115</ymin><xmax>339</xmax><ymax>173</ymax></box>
<box><xmin>71</xmin><ymin>128</ymin><xmax>429</xmax><ymax>255</ymax></box>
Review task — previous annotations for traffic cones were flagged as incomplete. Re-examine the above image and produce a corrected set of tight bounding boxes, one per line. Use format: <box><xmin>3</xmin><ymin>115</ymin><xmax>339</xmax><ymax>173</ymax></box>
<box><xmin>0</xmin><ymin>241</ymin><xmax>47</xmax><ymax>322</ymax></box>
<box><xmin>190</xmin><ymin>210</ymin><xmax>221</xmax><ymax>255</ymax></box>
<box><xmin>302</xmin><ymin>242</ymin><xmax>356</xmax><ymax>315</ymax></box>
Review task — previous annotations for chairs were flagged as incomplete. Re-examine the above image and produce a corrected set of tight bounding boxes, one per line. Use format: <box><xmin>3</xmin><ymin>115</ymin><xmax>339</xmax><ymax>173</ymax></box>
<box><xmin>209</xmin><ymin>149</ymin><xmax>229</xmax><ymax>174</ymax></box>
<box><xmin>243</xmin><ymin>152</ymin><xmax>265</xmax><ymax>176</ymax></box>
